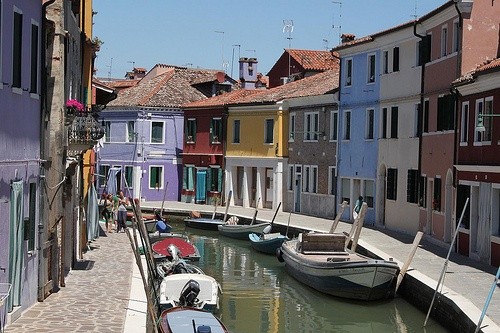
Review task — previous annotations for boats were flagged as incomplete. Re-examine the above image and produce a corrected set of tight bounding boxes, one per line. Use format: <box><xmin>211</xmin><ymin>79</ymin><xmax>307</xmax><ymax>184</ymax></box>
<box><xmin>127</xmin><ymin>212</ymin><xmax>165</xmax><ymax>224</ymax></box>
<box><xmin>184</xmin><ymin>218</ymin><xmax>400</xmax><ymax>302</ymax></box>
<box><xmin>151</xmin><ymin>237</ymin><xmax>227</xmax><ymax>333</ymax></box>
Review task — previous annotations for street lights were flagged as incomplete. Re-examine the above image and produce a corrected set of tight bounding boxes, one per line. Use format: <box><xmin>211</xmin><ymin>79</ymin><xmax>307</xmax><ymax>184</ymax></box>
<box><xmin>475</xmin><ymin>114</ymin><xmax>499</xmax><ymax>131</ymax></box>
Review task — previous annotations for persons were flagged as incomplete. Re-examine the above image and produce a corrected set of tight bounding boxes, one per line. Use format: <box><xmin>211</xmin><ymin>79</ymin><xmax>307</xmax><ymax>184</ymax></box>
<box><xmin>353</xmin><ymin>195</ymin><xmax>363</xmax><ymax>220</ymax></box>
<box><xmin>100</xmin><ymin>190</ymin><xmax>129</xmax><ymax>234</ymax></box>
